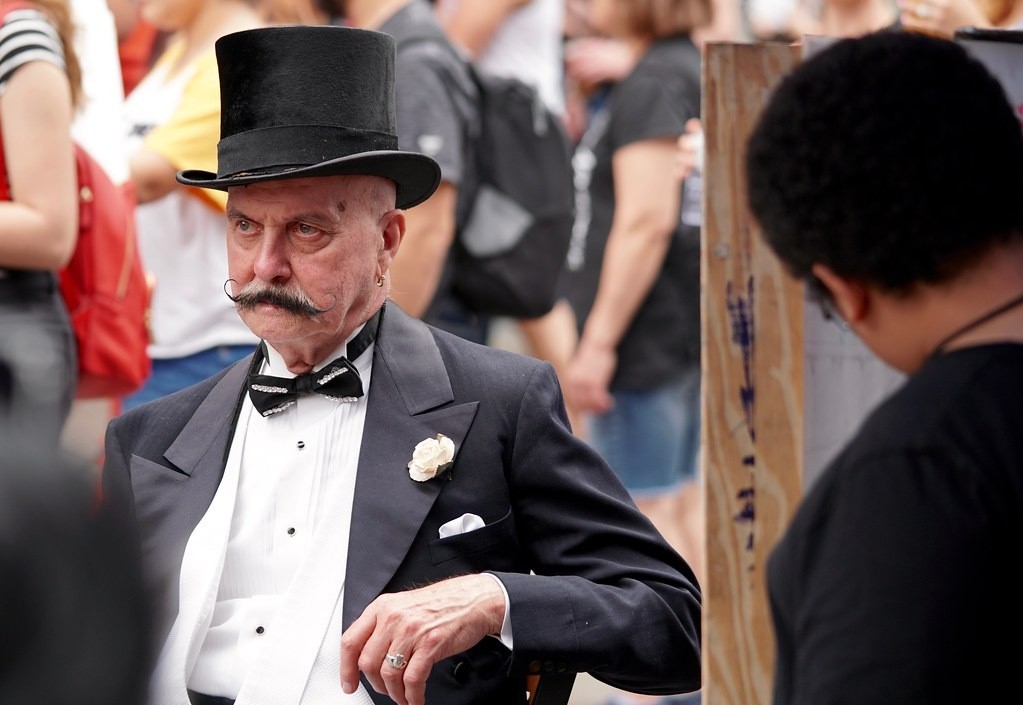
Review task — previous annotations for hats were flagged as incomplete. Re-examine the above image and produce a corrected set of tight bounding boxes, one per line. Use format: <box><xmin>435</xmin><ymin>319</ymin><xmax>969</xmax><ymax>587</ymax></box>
<box><xmin>175</xmin><ymin>26</ymin><xmax>441</xmax><ymax>210</ymax></box>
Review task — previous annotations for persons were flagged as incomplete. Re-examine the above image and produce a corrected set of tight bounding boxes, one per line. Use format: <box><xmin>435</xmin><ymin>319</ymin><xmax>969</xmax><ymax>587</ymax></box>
<box><xmin>740</xmin><ymin>29</ymin><xmax>1022</xmax><ymax>705</ymax></box>
<box><xmin>97</xmin><ymin>25</ymin><xmax>703</xmax><ymax>705</ymax></box>
<box><xmin>0</xmin><ymin>0</ymin><xmax>79</xmax><ymax>478</ymax></box>
<box><xmin>100</xmin><ymin>0</ymin><xmax>1023</xmax><ymax>705</ymax></box>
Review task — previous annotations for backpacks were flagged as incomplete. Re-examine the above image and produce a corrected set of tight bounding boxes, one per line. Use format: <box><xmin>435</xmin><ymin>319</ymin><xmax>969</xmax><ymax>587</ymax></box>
<box><xmin>0</xmin><ymin>0</ymin><xmax>156</xmax><ymax>400</ymax></box>
<box><xmin>398</xmin><ymin>35</ymin><xmax>576</xmax><ymax>318</ymax></box>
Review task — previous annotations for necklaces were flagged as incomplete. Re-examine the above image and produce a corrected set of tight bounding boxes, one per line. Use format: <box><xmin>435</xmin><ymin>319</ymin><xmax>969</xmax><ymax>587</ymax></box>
<box><xmin>934</xmin><ymin>295</ymin><xmax>1022</xmax><ymax>352</ymax></box>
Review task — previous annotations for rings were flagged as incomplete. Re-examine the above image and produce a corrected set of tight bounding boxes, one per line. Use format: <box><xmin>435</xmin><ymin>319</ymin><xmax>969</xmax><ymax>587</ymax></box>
<box><xmin>386</xmin><ymin>653</ymin><xmax>408</xmax><ymax>670</ymax></box>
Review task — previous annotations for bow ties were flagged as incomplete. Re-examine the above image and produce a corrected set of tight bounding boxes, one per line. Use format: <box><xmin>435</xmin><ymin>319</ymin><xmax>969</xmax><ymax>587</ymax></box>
<box><xmin>247</xmin><ymin>313</ymin><xmax>381</xmax><ymax>417</ymax></box>
